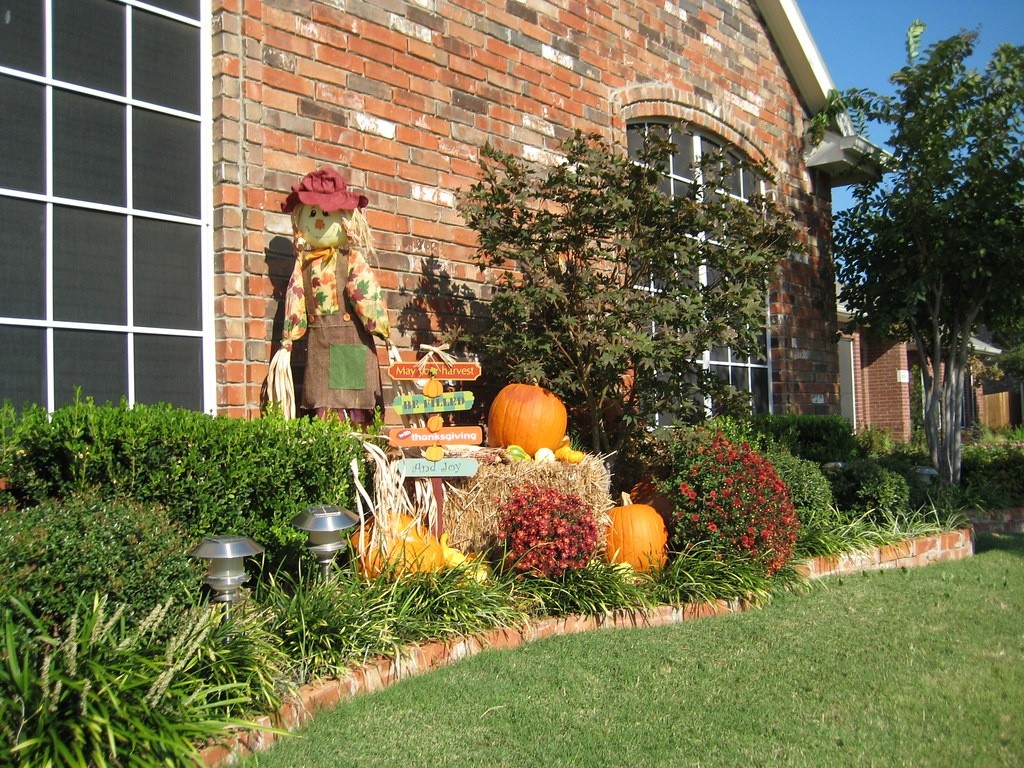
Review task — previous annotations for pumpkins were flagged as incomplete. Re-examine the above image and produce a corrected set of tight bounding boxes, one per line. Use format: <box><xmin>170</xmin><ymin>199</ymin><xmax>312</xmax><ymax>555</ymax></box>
<box><xmin>488</xmin><ymin>378</ymin><xmax>584</xmax><ymax>464</ymax></box>
<box><xmin>348</xmin><ymin>509</ymin><xmax>493</xmax><ymax>587</ymax></box>
<box><xmin>605</xmin><ymin>491</ymin><xmax>668</xmax><ymax>574</ymax></box>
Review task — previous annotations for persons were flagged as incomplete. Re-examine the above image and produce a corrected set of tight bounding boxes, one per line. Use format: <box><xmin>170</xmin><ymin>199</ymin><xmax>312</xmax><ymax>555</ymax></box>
<box><xmin>262</xmin><ymin>167</ymin><xmax>428</xmax><ymax>429</ymax></box>
